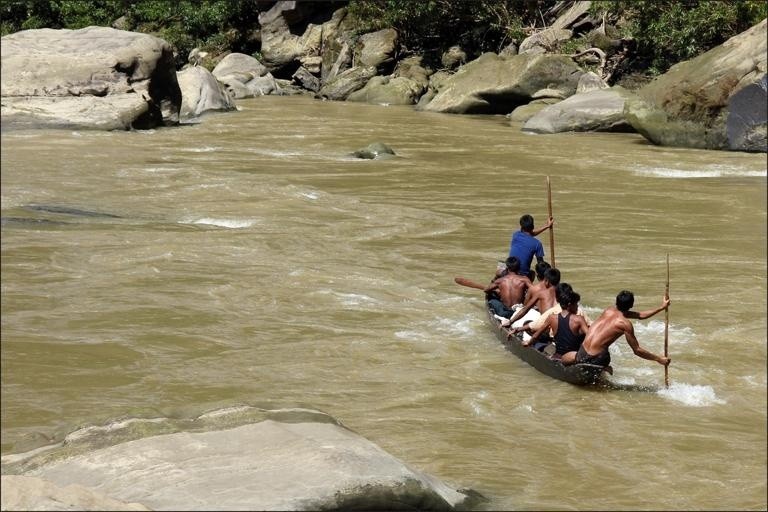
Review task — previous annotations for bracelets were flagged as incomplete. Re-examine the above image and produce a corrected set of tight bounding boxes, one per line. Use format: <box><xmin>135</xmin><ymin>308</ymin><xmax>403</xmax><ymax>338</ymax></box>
<box><xmin>509</xmin><ymin>320</ymin><xmax>511</xmax><ymax>323</ymax></box>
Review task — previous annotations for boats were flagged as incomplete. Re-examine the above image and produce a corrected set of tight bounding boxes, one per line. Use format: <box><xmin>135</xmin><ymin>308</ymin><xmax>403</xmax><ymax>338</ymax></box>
<box><xmin>486</xmin><ymin>262</ymin><xmax>618</xmax><ymax>387</ymax></box>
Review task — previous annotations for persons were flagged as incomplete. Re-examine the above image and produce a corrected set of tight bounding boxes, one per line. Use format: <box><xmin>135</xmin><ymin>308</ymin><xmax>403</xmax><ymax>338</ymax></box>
<box><xmin>524</xmin><ymin>290</ymin><xmax>588</xmax><ymax>360</ymax></box>
<box><xmin>507</xmin><ymin>283</ymin><xmax>594</xmax><ymax>356</ymax></box>
<box><xmin>562</xmin><ymin>290</ymin><xmax>670</xmax><ymax>367</ymax></box>
<box><xmin>484</xmin><ymin>257</ymin><xmax>532</xmax><ymax>318</ymax></box>
<box><xmin>498</xmin><ymin>267</ymin><xmax>560</xmax><ymax>328</ymax></box>
<box><xmin>497</xmin><ymin>215</ymin><xmax>553</xmax><ymax>282</ymax></box>
<box><xmin>524</xmin><ymin>262</ymin><xmax>551</xmax><ymax>310</ymax></box>
